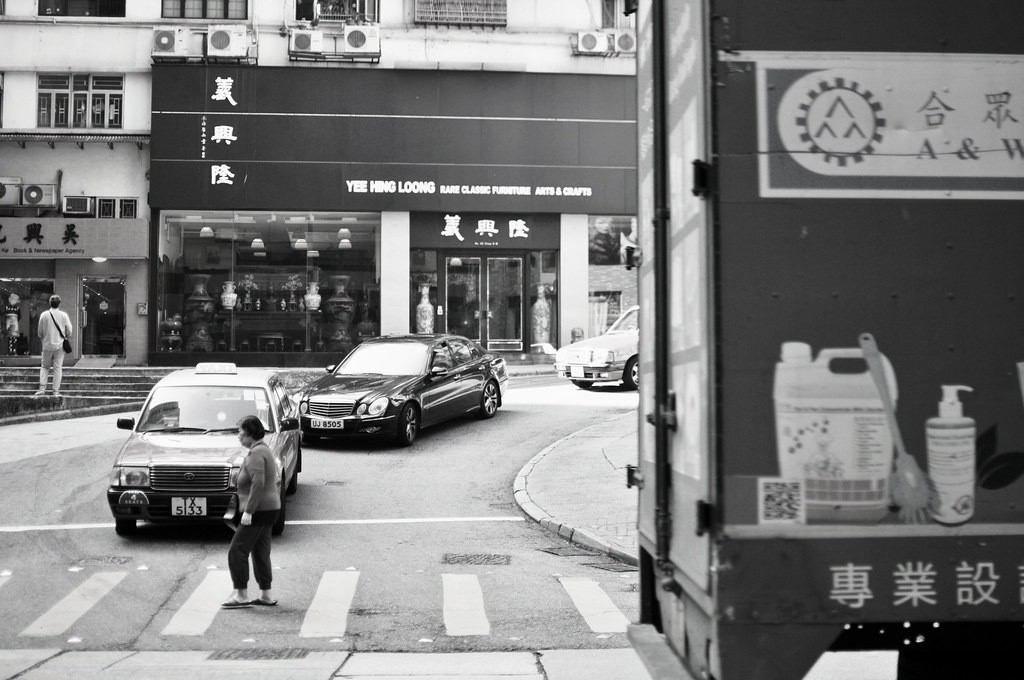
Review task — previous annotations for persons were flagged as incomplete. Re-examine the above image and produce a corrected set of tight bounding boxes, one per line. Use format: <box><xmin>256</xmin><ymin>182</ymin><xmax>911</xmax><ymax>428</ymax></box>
<box><xmin>220</xmin><ymin>417</ymin><xmax>282</xmax><ymax>609</ymax></box>
<box><xmin>35</xmin><ymin>296</ymin><xmax>73</xmax><ymax>395</ymax></box>
<box><xmin>588</xmin><ymin>215</ymin><xmax>620</xmax><ymax>265</ymax></box>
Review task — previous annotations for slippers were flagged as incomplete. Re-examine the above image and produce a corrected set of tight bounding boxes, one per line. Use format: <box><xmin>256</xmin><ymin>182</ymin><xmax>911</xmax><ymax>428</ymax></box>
<box><xmin>251</xmin><ymin>597</ymin><xmax>279</xmax><ymax>605</ymax></box>
<box><xmin>221</xmin><ymin>597</ymin><xmax>252</xmax><ymax>609</ymax></box>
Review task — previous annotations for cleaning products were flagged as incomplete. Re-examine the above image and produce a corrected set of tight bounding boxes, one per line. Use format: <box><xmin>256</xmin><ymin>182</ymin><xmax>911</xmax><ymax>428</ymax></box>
<box><xmin>924</xmin><ymin>380</ymin><xmax>978</xmax><ymax>527</ymax></box>
<box><xmin>772</xmin><ymin>339</ymin><xmax>898</xmax><ymax>522</ymax></box>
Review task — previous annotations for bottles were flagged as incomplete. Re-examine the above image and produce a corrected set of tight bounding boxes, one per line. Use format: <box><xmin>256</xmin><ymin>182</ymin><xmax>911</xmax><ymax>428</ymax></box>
<box><xmin>530</xmin><ymin>282</ymin><xmax>552</xmax><ymax>343</ymax></box>
<box><xmin>325</xmin><ymin>275</ymin><xmax>356</xmax><ymax>342</ymax></box>
<box><xmin>772</xmin><ymin>342</ymin><xmax>898</xmax><ymax>523</ymax></box>
<box><xmin>304</xmin><ymin>282</ymin><xmax>322</xmax><ymax>310</ymax></box>
<box><xmin>416</xmin><ymin>283</ymin><xmax>434</xmax><ymax>333</ymax></box>
<box><xmin>184</xmin><ymin>274</ymin><xmax>215</xmax><ymax>343</ymax></box>
<box><xmin>221</xmin><ymin>281</ymin><xmax>237</xmax><ymax>309</ymax></box>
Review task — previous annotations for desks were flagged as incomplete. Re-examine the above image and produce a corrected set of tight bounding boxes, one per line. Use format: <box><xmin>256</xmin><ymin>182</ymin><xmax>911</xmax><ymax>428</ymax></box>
<box><xmin>221</xmin><ymin>309</ymin><xmax>322</xmax><ymax>352</ymax></box>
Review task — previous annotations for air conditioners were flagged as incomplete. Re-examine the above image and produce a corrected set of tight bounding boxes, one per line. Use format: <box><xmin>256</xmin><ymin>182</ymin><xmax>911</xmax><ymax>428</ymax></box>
<box><xmin>577</xmin><ymin>31</ymin><xmax>608</xmax><ymax>51</ymax></box>
<box><xmin>207</xmin><ymin>25</ymin><xmax>247</xmax><ymax>56</ymax></box>
<box><xmin>22</xmin><ymin>185</ymin><xmax>56</xmax><ymax>207</ymax></box>
<box><xmin>614</xmin><ymin>32</ymin><xmax>636</xmax><ymax>52</ymax></box>
<box><xmin>344</xmin><ymin>26</ymin><xmax>379</xmax><ymax>53</ymax></box>
<box><xmin>0</xmin><ymin>176</ymin><xmax>22</xmax><ymax>205</ymax></box>
<box><xmin>290</xmin><ymin>30</ymin><xmax>322</xmax><ymax>52</ymax></box>
<box><xmin>151</xmin><ymin>27</ymin><xmax>190</xmax><ymax>56</ymax></box>
<box><xmin>63</xmin><ymin>197</ymin><xmax>94</xmax><ymax>215</ymax></box>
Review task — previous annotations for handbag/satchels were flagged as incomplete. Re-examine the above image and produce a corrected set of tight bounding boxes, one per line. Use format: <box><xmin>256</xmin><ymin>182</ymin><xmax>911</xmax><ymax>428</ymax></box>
<box><xmin>62</xmin><ymin>339</ymin><xmax>72</xmax><ymax>354</ymax></box>
<box><xmin>222</xmin><ymin>493</ymin><xmax>240</xmax><ymax>532</ymax></box>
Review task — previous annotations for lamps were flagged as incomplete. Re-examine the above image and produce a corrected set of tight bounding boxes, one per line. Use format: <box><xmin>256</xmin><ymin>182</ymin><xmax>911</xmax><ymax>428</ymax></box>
<box><xmin>200</xmin><ymin>227</ymin><xmax>214</xmax><ymax>237</ymax></box>
<box><xmin>251</xmin><ymin>237</ymin><xmax>264</xmax><ymax>248</ymax></box>
<box><xmin>337</xmin><ymin>227</ymin><xmax>351</xmax><ymax>238</ymax></box>
<box><xmin>339</xmin><ymin>239</ymin><xmax>352</xmax><ymax>248</ymax></box>
<box><xmin>295</xmin><ymin>238</ymin><xmax>307</xmax><ymax>248</ymax></box>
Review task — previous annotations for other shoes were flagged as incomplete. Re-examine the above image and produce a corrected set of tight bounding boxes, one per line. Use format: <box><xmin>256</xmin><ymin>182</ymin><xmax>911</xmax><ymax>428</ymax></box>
<box><xmin>35</xmin><ymin>391</ymin><xmax>45</xmax><ymax>395</ymax></box>
<box><xmin>53</xmin><ymin>392</ymin><xmax>63</xmax><ymax>396</ymax></box>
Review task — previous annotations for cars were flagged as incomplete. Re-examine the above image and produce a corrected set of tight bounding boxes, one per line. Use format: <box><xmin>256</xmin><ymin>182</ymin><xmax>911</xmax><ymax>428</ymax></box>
<box><xmin>298</xmin><ymin>332</ymin><xmax>509</xmax><ymax>448</ymax></box>
<box><xmin>553</xmin><ymin>305</ymin><xmax>638</xmax><ymax>391</ymax></box>
<box><xmin>106</xmin><ymin>361</ymin><xmax>303</xmax><ymax>537</ymax></box>
